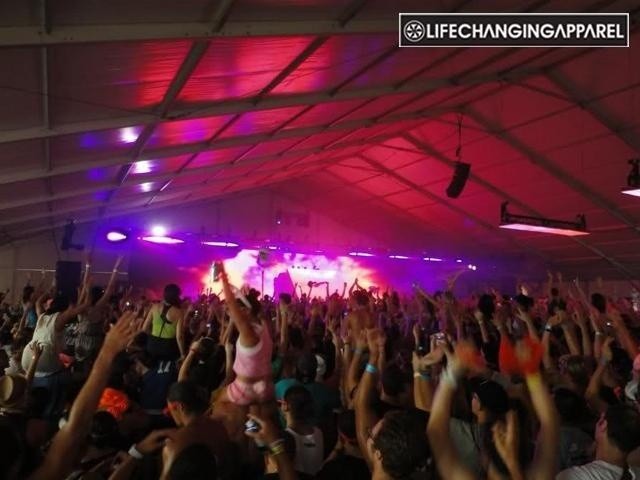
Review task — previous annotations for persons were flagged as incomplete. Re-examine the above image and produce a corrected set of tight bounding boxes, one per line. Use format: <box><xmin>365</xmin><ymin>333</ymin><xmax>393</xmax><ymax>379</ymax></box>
<box><xmin>1</xmin><ymin>253</ymin><xmax>640</xmax><ymax>479</ymax></box>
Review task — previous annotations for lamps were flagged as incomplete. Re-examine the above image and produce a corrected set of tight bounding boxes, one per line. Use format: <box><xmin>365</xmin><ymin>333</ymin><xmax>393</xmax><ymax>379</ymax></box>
<box><xmin>494</xmin><ymin>199</ymin><xmax>591</xmax><ymax>239</ymax></box>
